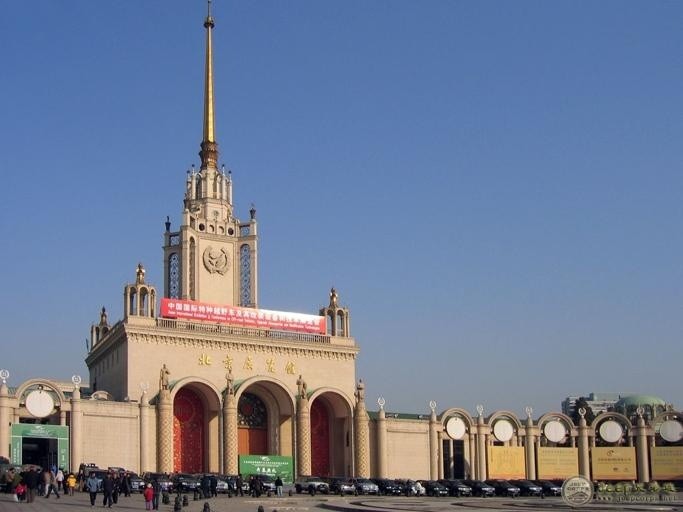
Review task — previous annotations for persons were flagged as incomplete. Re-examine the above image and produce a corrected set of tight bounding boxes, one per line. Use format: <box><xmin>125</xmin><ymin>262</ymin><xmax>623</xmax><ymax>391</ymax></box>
<box><xmin>296</xmin><ymin>375</ymin><xmax>306</xmax><ymax>399</ymax></box>
<box><xmin>165</xmin><ymin>470</ymin><xmax>183</xmax><ymax>497</ymax></box>
<box><xmin>196</xmin><ymin>475</ymin><xmax>217</xmax><ymax>499</ymax></box>
<box><xmin>414</xmin><ymin>481</ymin><xmax>421</xmax><ymax>497</ymax></box>
<box><xmin>144</xmin><ymin>483</ymin><xmax>154</xmax><ymax>510</ymax></box>
<box><xmin>275</xmin><ymin>477</ymin><xmax>283</xmax><ymax>496</ymax></box>
<box><xmin>357</xmin><ymin>380</ymin><xmax>364</xmax><ymax>401</ymax></box>
<box><xmin>405</xmin><ymin>480</ymin><xmax>412</xmax><ymax>497</ymax></box>
<box><xmin>226</xmin><ymin>368</ymin><xmax>235</xmax><ymax>394</ymax></box>
<box><xmin>160</xmin><ymin>364</ymin><xmax>170</xmax><ymax>390</ymax></box>
<box><xmin>152</xmin><ymin>479</ymin><xmax>162</xmax><ymax>511</ymax></box>
<box><xmin>77</xmin><ymin>469</ymin><xmax>85</xmax><ymax>493</ymax></box>
<box><xmin>17</xmin><ymin>464</ymin><xmax>76</xmax><ymax>504</ymax></box>
<box><xmin>226</xmin><ymin>474</ymin><xmax>265</xmax><ymax>498</ymax></box>
<box><xmin>84</xmin><ymin>468</ymin><xmax>132</xmax><ymax>507</ymax></box>
<box><xmin>6</xmin><ymin>467</ymin><xmax>15</xmax><ymax>492</ymax></box>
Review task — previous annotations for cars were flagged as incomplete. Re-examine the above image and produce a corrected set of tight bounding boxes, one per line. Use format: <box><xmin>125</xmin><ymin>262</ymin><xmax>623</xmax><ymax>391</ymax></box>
<box><xmin>294</xmin><ymin>475</ymin><xmax>426</xmax><ymax>497</ymax></box>
<box><xmin>172</xmin><ymin>472</ymin><xmax>278</xmax><ymax>493</ymax></box>
<box><xmin>83</xmin><ymin>468</ymin><xmax>174</xmax><ymax>494</ymax></box>
<box><xmin>417</xmin><ymin>478</ymin><xmax>561</xmax><ymax>498</ymax></box>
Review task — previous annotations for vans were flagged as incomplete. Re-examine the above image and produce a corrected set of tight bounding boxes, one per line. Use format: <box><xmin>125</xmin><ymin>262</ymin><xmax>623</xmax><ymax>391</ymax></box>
<box><xmin>0</xmin><ymin>465</ymin><xmax>23</xmax><ymax>493</ymax></box>
